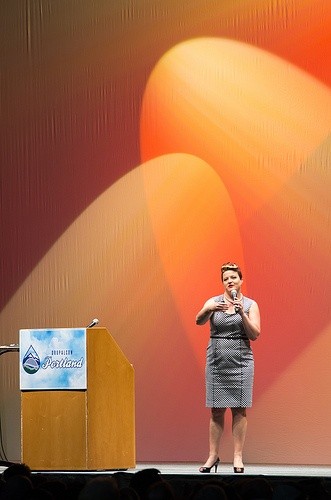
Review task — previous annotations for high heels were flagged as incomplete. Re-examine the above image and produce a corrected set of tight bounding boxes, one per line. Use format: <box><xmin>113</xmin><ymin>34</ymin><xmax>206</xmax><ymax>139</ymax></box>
<box><xmin>234</xmin><ymin>467</ymin><xmax>244</xmax><ymax>473</ymax></box>
<box><xmin>199</xmin><ymin>456</ymin><xmax>220</xmax><ymax>473</ymax></box>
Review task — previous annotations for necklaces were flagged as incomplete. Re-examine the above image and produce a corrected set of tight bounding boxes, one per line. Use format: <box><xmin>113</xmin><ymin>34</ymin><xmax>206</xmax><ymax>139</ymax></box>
<box><xmin>224</xmin><ymin>292</ymin><xmax>243</xmax><ymax>303</ymax></box>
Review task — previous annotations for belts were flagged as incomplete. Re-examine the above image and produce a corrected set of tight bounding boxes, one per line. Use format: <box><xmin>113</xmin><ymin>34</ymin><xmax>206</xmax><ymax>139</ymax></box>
<box><xmin>210</xmin><ymin>336</ymin><xmax>249</xmax><ymax>340</ymax></box>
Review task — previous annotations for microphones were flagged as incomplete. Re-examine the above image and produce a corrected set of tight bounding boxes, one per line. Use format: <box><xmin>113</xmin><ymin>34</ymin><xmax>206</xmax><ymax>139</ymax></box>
<box><xmin>231</xmin><ymin>289</ymin><xmax>240</xmax><ymax>314</ymax></box>
<box><xmin>87</xmin><ymin>318</ymin><xmax>100</xmax><ymax>328</ymax></box>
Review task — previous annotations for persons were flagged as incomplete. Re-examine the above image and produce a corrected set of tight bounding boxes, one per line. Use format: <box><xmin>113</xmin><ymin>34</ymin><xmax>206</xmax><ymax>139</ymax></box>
<box><xmin>0</xmin><ymin>462</ymin><xmax>331</xmax><ymax>500</ymax></box>
<box><xmin>196</xmin><ymin>261</ymin><xmax>261</xmax><ymax>473</ymax></box>
